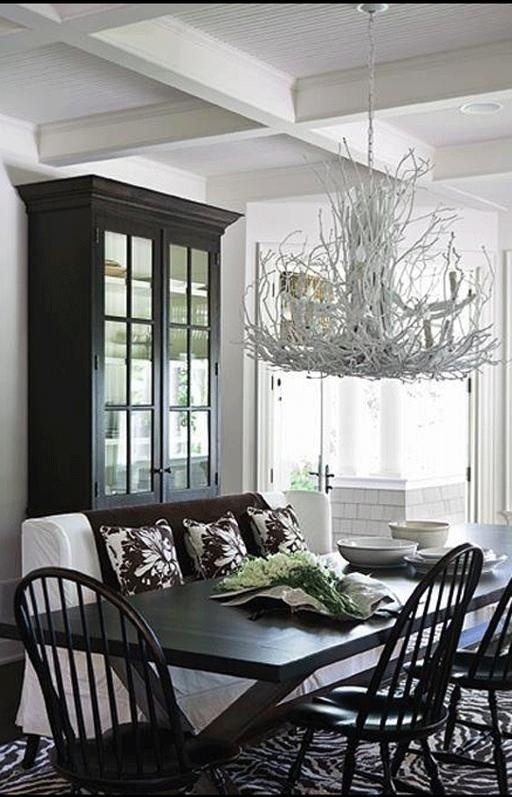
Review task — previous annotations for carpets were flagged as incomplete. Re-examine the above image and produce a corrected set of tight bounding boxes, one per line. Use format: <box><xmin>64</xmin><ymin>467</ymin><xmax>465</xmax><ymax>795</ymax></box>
<box><xmin>0</xmin><ymin>606</ymin><xmax>512</xmax><ymax>797</ymax></box>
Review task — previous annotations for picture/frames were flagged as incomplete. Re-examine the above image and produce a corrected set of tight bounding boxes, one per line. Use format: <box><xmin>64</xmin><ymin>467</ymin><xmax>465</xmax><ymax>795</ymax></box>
<box><xmin>280</xmin><ymin>272</ymin><xmax>334</xmax><ymax>346</ymax></box>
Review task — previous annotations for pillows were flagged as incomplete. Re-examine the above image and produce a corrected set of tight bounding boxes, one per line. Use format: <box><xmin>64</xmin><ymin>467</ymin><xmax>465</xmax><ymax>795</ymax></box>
<box><xmin>181</xmin><ymin>508</ymin><xmax>249</xmax><ymax>581</ymax></box>
<box><xmin>247</xmin><ymin>504</ymin><xmax>309</xmax><ymax>560</ymax></box>
<box><xmin>98</xmin><ymin>517</ymin><xmax>185</xmax><ymax>596</ymax></box>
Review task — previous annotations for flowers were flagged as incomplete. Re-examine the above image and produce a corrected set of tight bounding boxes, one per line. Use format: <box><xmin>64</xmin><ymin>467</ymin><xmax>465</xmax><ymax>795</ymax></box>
<box><xmin>213</xmin><ymin>544</ymin><xmax>364</xmax><ymax>618</ymax></box>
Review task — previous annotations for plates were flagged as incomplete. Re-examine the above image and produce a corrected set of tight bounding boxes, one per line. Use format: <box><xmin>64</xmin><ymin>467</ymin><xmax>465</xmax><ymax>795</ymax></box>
<box><xmin>169</xmin><ymin>277</ymin><xmax>207</xmax><ymax>289</ymax></box>
<box><xmin>418</xmin><ymin>546</ymin><xmax>492</xmax><ymax>559</ymax></box>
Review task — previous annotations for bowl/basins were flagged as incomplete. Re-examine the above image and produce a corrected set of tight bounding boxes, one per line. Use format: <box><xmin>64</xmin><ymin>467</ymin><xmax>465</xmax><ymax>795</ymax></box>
<box><xmin>402</xmin><ymin>554</ymin><xmax>509</xmax><ymax>576</ymax></box>
<box><xmin>105</xmin><ymin>260</ymin><xmax>127</xmax><ymax>277</ymax></box>
<box><xmin>388</xmin><ymin>518</ymin><xmax>452</xmax><ymax>551</ymax></box>
<box><xmin>336</xmin><ymin>536</ymin><xmax>418</xmax><ymax>569</ymax></box>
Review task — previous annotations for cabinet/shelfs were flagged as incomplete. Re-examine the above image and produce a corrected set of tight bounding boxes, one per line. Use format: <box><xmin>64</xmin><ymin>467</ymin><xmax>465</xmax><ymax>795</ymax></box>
<box><xmin>14</xmin><ymin>175</ymin><xmax>245</xmax><ymax>518</ymax></box>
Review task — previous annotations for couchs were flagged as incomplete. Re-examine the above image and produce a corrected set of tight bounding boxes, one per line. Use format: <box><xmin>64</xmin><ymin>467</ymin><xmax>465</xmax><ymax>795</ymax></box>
<box><xmin>15</xmin><ymin>489</ymin><xmax>405</xmax><ymax>770</ymax></box>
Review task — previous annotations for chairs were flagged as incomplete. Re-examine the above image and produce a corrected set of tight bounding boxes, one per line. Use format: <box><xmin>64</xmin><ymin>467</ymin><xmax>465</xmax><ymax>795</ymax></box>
<box><xmin>13</xmin><ymin>568</ymin><xmax>242</xmax><ymax>797</ymax></box>
<box><xmin>403</xmin><ymin>578</ymin><xmax>512</xmax><ymax>795</ymax></box>
<box><xmin>287</xmin><ymin>543</ymin><xmax>484</xmax><ymax>796</ymax></box>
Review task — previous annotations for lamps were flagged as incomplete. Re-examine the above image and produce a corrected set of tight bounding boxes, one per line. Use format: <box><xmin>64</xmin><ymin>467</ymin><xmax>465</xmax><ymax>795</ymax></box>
<box><xmin>230</xmin><ymin>4</ymin><xmax>511</xmax><ymax>384</ymax></box>
